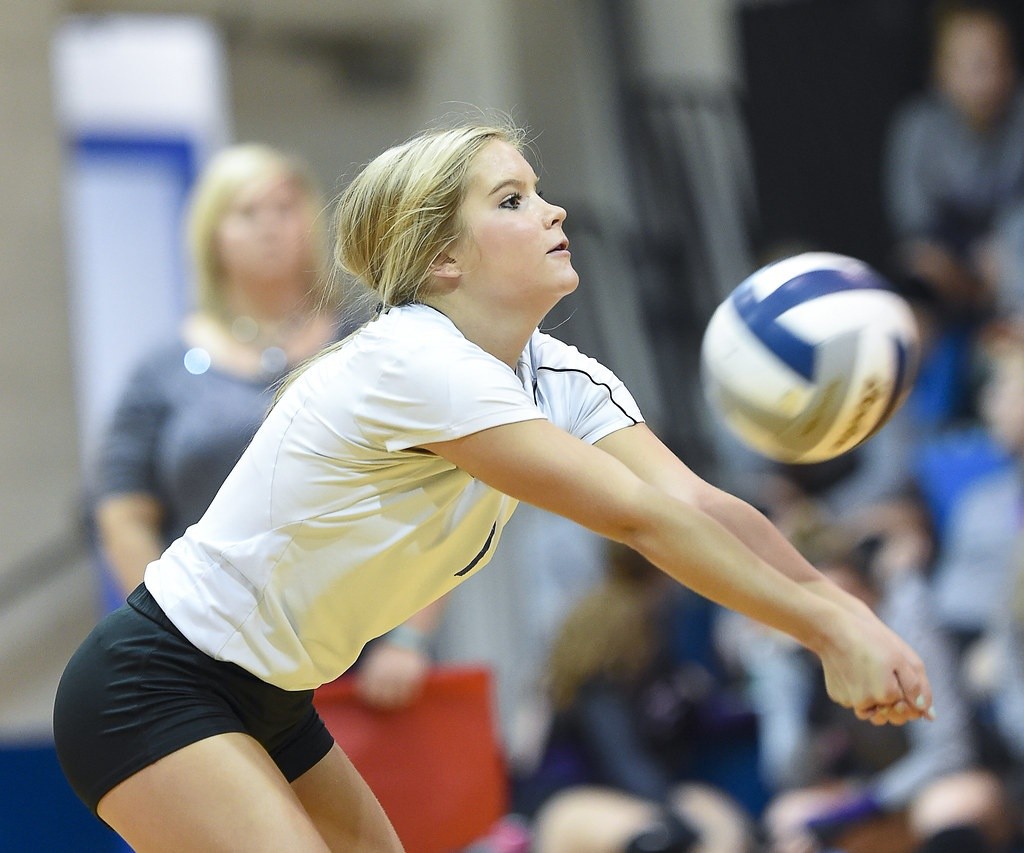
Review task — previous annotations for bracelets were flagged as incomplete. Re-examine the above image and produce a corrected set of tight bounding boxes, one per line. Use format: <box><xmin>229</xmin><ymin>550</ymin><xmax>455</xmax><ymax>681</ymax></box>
<box><xmin>387</xmin><ymin>628</ymin><xmax>441</xmax><ymax>653</ymax></box>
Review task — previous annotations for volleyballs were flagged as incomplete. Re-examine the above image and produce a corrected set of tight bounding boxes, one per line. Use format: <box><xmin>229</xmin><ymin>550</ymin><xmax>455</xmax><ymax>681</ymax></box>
<box><xmin>698</xmin><ymin>250</ymin><xmax>918</xmax><ymax>465</ymax></box>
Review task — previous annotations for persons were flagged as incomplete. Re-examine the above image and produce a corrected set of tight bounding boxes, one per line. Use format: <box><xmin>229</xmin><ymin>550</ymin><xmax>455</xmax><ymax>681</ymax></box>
<box><xmin>87</xmin><ymin>143</ymin><xmax>373</xmax><ymax>602</ymax></box>
<box><xmin>349</xmin><ymin>0</ymin><xmax>1024</xmax><ymax>853</ymax></box>
<box><xmin>52</xmin><ymin>124</ymin><xmax>936</xmax><ymax>853</ymax></box>
